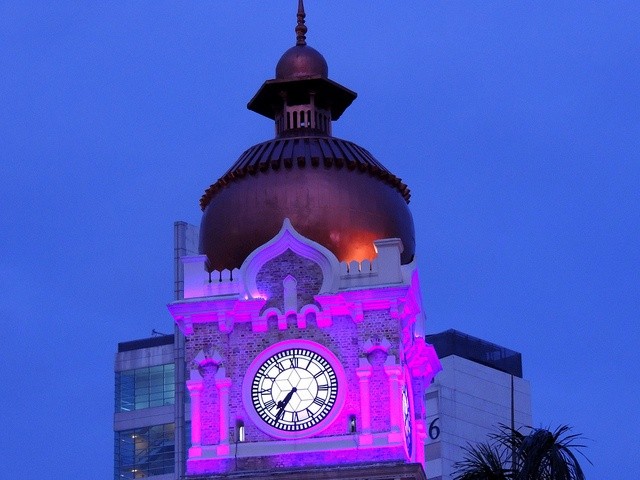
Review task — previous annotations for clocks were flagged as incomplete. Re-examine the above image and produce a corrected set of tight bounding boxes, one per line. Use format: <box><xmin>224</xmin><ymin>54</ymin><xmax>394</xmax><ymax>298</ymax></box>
<box><xmin>402</xmin><ymin>384</ymin><xmax>413</xmax><ymax>457</ymax></box>
<box><xmin>252</xmin><ymin>348</ymin><xmax>337</xmax><ymax>432</ymax></box>
